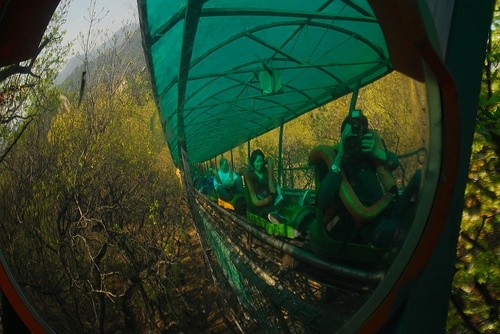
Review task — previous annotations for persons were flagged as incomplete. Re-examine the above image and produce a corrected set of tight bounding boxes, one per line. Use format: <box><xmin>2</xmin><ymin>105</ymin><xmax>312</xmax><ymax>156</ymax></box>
<box><xmin>244</xmin><ymin>149</ymin><xmax>284</xmax><ymax>225</ymax></box>
<box><xmin>193</xmin><ymin>168</ymin><xmax>214</xmax><ymax>195</ymax></box>
<box><xmin>316</xmin><ymin>110</ymin><xmax>426</xmax><ymax>247</ymax></box>
<box><xmin>213</xmin><ymin>158</ymin><xmax>244</xmax><ymax>203</ymax></box>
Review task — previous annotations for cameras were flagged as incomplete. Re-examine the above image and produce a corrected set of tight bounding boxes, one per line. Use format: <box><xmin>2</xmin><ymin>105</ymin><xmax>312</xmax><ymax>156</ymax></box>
<box><xmin>345</xmin><ymin>109</ymin><xmax>369</xmax><ymax>160</ymax></box>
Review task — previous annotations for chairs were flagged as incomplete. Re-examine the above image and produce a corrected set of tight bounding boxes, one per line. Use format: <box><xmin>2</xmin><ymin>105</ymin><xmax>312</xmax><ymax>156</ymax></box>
<box><xmin>204</xmin><ymin>129</ymin><xmax>420</xmax><ymax>277</ymax></box>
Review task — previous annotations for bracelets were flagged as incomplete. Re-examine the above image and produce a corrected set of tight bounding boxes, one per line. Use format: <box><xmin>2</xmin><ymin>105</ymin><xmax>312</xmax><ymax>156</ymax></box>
<box><xmin>330</xmin><ymin>165</ymin><xmax>342</xmax><ymax>173</ymax></box>
